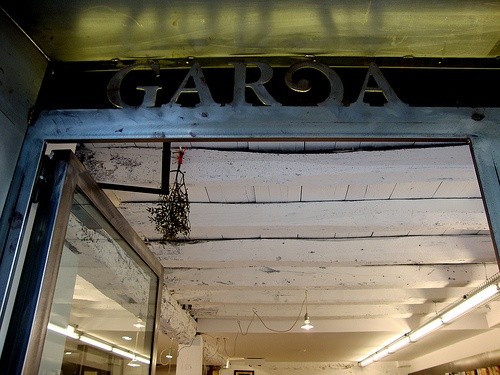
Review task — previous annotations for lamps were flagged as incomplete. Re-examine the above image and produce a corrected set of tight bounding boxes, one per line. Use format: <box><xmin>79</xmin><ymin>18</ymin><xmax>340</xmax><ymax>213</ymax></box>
<box><xmin>301</xmin><ymin>313</ymin><xmax>313</xmax><ymax>330</ymax></box>
<box><xmin>127</xmin><ymin>352</ymin><xmax>141</xmax><ymax>366</ymax></box>
<box><xmin>356</xmin><ymin>283</ymin><xmax>500</xmax><ymax>369</ymax></box>
